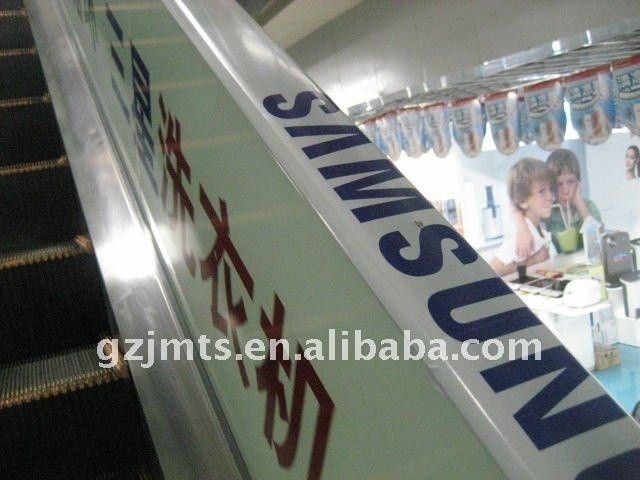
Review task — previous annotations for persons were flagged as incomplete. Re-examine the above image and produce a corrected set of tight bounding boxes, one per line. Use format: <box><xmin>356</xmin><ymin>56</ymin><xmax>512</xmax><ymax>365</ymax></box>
<box><xmin>611</xmin><ymin>146</ymin><xmax>640</xmax><ymax>227</ymax></box>
<box><xmin>512</xmin><ymin>149</ymin><xmax>602</xmax><ymax>259</ymax></box>
<box><xmin>491</xmin><ymin>157</ymin><xmax>559</xmax><ymax>278</ymax></box>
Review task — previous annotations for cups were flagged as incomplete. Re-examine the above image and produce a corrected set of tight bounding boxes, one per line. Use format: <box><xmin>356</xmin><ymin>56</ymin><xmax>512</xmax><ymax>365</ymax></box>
<box><xmin>553</xmin><ymin>229</ymin><xmax>578</xmax><ymax>254</ymax></box>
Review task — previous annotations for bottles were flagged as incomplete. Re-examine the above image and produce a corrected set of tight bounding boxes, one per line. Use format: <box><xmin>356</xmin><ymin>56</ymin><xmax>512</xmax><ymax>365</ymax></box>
<box><xmin>480</xmin><ymin>184</ymin><xmax>505</xmax><ymax>242</ymax></box>
